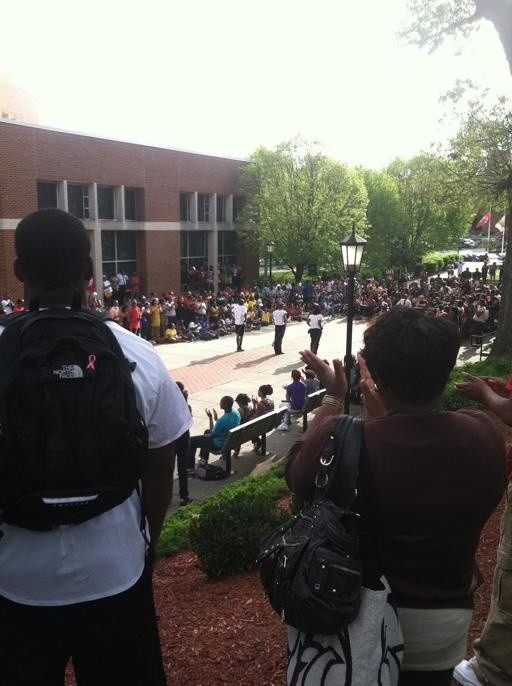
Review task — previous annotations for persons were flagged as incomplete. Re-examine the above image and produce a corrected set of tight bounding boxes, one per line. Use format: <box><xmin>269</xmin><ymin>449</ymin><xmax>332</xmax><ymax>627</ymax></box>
<box><xmin>0</xmin><ymin>207</ymin><xmax>193</xmax><ymax>685</ymax></box>
<box><xmin>284</xmin><ymin>303</ymin><xmax>507</xmax><ymax>685</ymax></box>
<box><xmin>90</xmin><ymin>259</ymin><xmax>504</xmax><ymax>480</ymax></box>
<box><xmin>0</xmin><ymin>292</ymin><xmax>25</xmax><ymax>336</ymax></box>
<box><xmin>452</xmin><ymin>369</ymin><xmax>512</xmax><ymax>686</ymax></box>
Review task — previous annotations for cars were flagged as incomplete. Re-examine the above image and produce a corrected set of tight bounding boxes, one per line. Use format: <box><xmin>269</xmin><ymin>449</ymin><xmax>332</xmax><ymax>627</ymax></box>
<box><xmin>457</xmin><ymin>235</ymin><xmax>488</xmax><ymax>248</ymax></box>
<box><xmin>497</xmin><ymin>250</ymin><xmax>507</xmax><ymax>261</ymax></box>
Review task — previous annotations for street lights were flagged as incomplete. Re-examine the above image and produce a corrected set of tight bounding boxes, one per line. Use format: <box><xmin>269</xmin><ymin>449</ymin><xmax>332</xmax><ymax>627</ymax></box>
<box><xmin>339</xmin><ymin>217</ymin><xmax>369</xmax><ymax>414</ymax></box>
<box><xmin>266</xmin><ymin>238</ymin><xmax>276</xmax><ymax>282</ymax></box>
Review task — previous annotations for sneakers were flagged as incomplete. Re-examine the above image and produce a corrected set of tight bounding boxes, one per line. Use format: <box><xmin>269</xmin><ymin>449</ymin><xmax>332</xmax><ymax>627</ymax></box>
<box><xmin>452</xmin><ymin>656</ymin><xmax>483</xmax><ymax>686</ymax></box>
<box><xmin>179</xmin><ymin>496</ymin><xmax>193</xmax><ymax>506</ymax></box>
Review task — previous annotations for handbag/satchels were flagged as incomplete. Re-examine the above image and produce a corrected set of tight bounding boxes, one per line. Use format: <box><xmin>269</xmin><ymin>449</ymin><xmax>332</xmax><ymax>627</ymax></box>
<box><xmin>285</xmin><ymin>560</ymin><xmax>407</xmax><ymax>685</ymax></box>
<box><xmin>350</xmin><ymin>389</ymin><xmax>361</xmax><ymax>405</ymax></box>
<box><xmin>254</xmin><ymin>415</ymin><xmax>370</xmax><ymax>638</ymax></box>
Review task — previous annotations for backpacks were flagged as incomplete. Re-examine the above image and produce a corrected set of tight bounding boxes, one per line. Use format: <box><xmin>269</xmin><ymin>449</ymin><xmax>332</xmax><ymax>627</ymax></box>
<box><xmin>0</xmin><ymin>309</ymin><xmax>149</xmax><ymax>533</ymax></box>
<box><xmin>196</xmin><ymin>463</ymin><xmax>225</xmax><ymax>481</ymax></box>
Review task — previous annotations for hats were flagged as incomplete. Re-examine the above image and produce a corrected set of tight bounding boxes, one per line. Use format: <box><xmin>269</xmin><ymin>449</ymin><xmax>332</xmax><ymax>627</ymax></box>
<box><xmin>278</xmin><ymin>302</ymin><xmax>286</xmax><ymax>306</ymax></box>
<box><xmin>303</xmin><ymin>369</ymin><xmax>316</xmax><ymax>378</ymax></box>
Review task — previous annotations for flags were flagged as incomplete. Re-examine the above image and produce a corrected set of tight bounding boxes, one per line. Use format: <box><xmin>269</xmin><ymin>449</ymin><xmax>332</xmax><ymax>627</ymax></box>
<box><xmin>476</xmin><ymin>211</ymin><xmax>491</xmax><ymax>228</ymax></box>
<box><xmin>494</xmin><ymin>215</ymin><xmax>505</xmax><ymax>232</ymax></box>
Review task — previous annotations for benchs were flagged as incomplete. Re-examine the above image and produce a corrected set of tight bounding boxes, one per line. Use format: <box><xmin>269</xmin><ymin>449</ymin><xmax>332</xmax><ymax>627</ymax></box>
<box><xmin>282</xmin><ymin>388</ymin><xmax>327</xmax><ymax>431</ymax></box>
<box><xmin>204</xmin><ymin>407</ymin><xmax>288</xmax><ymax>475</ymax></box>
